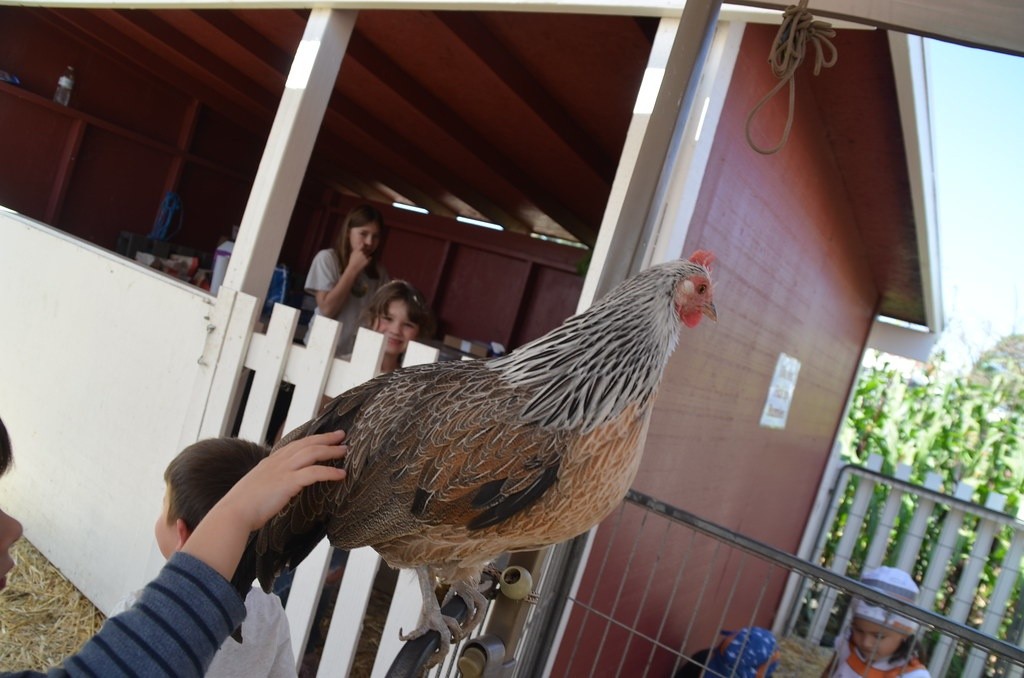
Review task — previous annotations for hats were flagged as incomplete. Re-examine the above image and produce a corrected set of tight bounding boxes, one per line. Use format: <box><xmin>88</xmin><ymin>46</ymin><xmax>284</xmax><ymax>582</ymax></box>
<box><xmin>853</xmin><ymin>565</ymin><xmax>920</xmax><ymax>636</ymax></box>
<box><xmin>715</xmin><ymin>627</ymin><xmax>780</xmax><ymax>678</ymax></box>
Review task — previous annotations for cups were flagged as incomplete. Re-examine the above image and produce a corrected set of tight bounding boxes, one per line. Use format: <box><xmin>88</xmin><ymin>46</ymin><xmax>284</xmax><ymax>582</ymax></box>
<box><xmin>135</xmin><ymin>251</ymin><xmax>155</xmax><ymax>266</ymax></box>
<box><xmin>170</xmin><ymin>254</ymin><xmax>198</xmax><ymax>275</ymax></box>
<box><xmin>189</xmin><ymin>268</ymin><xmax>211</xmax><ymax>292</ymax></box>
<box><xmin>167</xmin><ymin>260</ymin><xmax>182</xmax><ymax>276</ymax></box>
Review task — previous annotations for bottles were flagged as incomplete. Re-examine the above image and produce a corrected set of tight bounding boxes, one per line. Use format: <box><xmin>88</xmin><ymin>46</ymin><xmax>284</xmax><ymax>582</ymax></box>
<box><xmin>53</xmin><ymin>65</ymin><xmax>74</xmax><ymax>107</ymax></box>
<box><xmin>209</xmin><ymin>240</ymin><xmax>235</xmax><ymax>297</ymax></box>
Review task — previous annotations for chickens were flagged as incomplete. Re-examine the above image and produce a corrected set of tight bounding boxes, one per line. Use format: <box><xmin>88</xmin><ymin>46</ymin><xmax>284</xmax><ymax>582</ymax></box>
<box><xmin>256</xmin><ymin>249</ymin><xmax>722</xmax><ymax>671</ymax></box>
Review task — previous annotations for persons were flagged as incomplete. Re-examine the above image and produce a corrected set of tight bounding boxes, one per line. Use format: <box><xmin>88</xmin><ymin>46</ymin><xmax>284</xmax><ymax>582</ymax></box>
<box><xmin>255</xmin><ymin>204</ymin><xmax>390</xmax><ymax>447</ymax></box>
<box><xmin>98</xmin><ymin>437</ymin><xmax>298</xmax><ymax>678</ymax></box>
<box><xmin>271</xmin><ymin>279</ymin><xmax>434</xmax><ymax>653</ymax></box>
<box><xmin>834</xmin><ymin>566</ymin><xmax>932</xmax><ymax>678</ymax></box>
<box><xmin>0</xmin><ymin>417</ymin><xmax>349</xmax><ymax>678</ymax></box>
